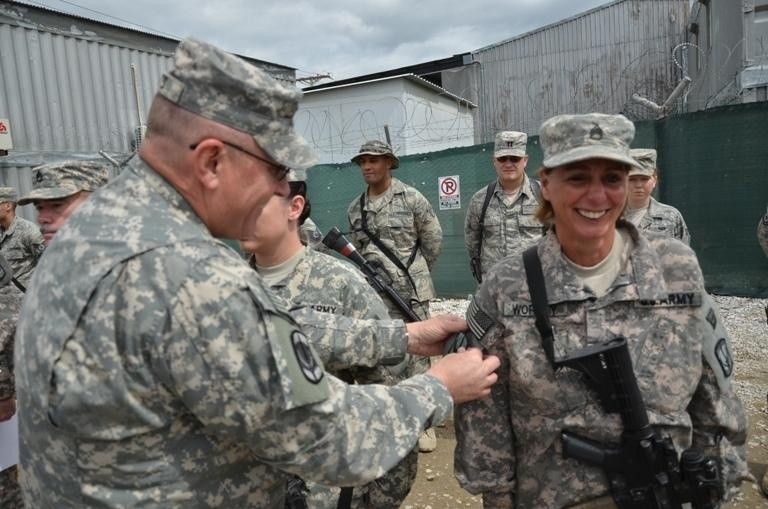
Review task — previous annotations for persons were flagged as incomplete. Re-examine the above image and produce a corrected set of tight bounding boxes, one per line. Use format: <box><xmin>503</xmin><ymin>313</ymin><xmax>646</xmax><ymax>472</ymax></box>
<box><xmin>235</xmin><ymin>136</ymin><xmax>419</xmax><ymax>509</ymax></box>
<box><xmin>14</xmin><ymin>37</ymin><xmax>501</xmax><ymax>509</ymax></box>
<box><xmin>0</xmin><ymin>186</ymin><xmax>46</xmax><ymax>293</ymax></box>
<box><xmin>347</xmin><ymin>140</ymin><xmax>443</xmax><ymax>454</ymax></box>
<box><xmin>757</xmin><ymin>211</ymin><xmax>768</xmax><ymax>260</ymax></box>
<box><xmin>11</xmin><ymin>161</ymin><xmax>110</xmax><ymax>245</ymax></box>
<box><xmin>297</xmin><ymin>215</ymin><xmax>332</xmax><ymax>256</ymax></box>
<box><xmin>619</xmin><ymin>148</ymin><xmax>691</xmax><ymax>248</ymax></box>
<box><xmin>0</xmin><ymin>251</ymin><xmax>26</xmax><ymax>508</ymax></box>
<box><xmin>464</xmin><ymin>130</ymin><xmax>553</xmax><ymax>285</ymax></box>
<box><xmin>442</xmin><ymin>112</ymin><xmax>768</xmax><ymax>509</ymax></box>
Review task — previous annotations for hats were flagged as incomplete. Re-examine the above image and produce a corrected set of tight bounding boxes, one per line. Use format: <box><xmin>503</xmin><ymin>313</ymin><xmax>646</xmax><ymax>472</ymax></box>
<box><xmin>351</xmin><ymin>140</ymin><xmax>399</xmax><ymax>169</ymax></box>
<box><xmin>540</xmin><ymin>111</ymin><xmax>645</xmax><ymax>172</ymax></box>
<box><xmin>0</xmin><ymin>185</ymin><xmax>16</xmax><ymax>204</ymax></box>
<box><xmin>287</xmin><ymin>168</ymin><xmax>310</xmax><ymax>182</ymax></box>
<box><xmin>15</xmin><ymin>157</ymin><xmax>110</xmax><ymax>207</ymax></box>
<box><xmin>159</xmin><ymin>34</ymin><xmax>319</xmax><ymax>170</ymax></box>
<box><xmin>629</xmin><ymin>147</ymin><xmax>657</xmax><ymax>178</ymax></box>
<box><xmin>494</xmin><ymin>130</ymin><xmax>528</xmax><ymax>160</ymax></box>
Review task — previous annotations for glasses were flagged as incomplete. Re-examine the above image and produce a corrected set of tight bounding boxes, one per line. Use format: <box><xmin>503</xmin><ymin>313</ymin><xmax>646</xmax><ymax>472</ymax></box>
<box><xmin>188</xmin><ymin>136</ymin><xmax>293</xmax><ymax>181</ymax></box>
<box><xmin>496</xmin><ymin>156</ymin><xmax>522</xmax><ymax>163</ymax></box>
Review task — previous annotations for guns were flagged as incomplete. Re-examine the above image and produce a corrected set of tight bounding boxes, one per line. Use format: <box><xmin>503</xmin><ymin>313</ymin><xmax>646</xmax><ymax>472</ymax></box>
<box><xmin>322</xmin><ymin>226</ymin><xmax>423</xmax><ymax>323</ymax></box>
<box><xmin>553</xmin><ymin>335</ymin><xmax>725</xmax><ymax>509</ymax></box>
